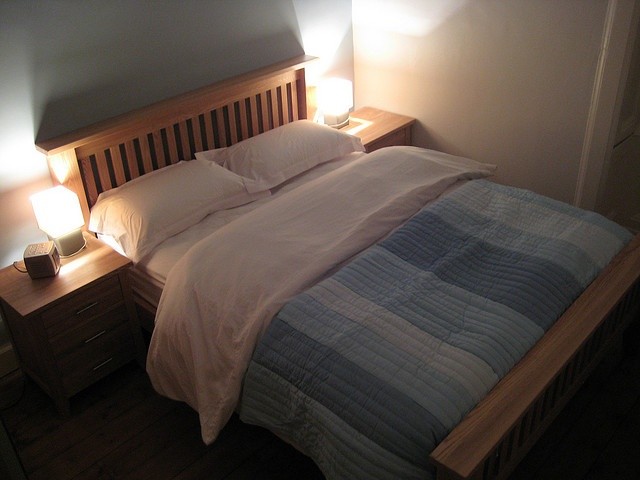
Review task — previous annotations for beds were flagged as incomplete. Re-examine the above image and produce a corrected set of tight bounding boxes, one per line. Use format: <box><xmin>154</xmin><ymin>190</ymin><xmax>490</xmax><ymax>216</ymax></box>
<box><xmin>34</xmin><ymin>54</ymin><xmax>640</xmax><ymax>476</ymax></box>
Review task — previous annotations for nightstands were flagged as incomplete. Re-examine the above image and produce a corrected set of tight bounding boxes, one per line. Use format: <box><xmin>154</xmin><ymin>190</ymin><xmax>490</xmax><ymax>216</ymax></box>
<box><xmin>314</xmin><ymin>105</ymin><xmax>418</xmax><ymax>155</ymax></box>
<box><xmin>0</xmin><ymin>230</ymin><xmax>147</xmax><ymax>416</ymax></box>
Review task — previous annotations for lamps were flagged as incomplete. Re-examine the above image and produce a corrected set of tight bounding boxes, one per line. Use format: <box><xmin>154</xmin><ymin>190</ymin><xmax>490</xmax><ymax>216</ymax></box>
<box><xmin>29</xmin><ymin>183</ymin><xmax>89</xmax><ymax>259</ymax></box>
<box><xmin>314</xmin><ymin>75</ymin><xmax>354</xmax><ymax>130</ymax></box>
